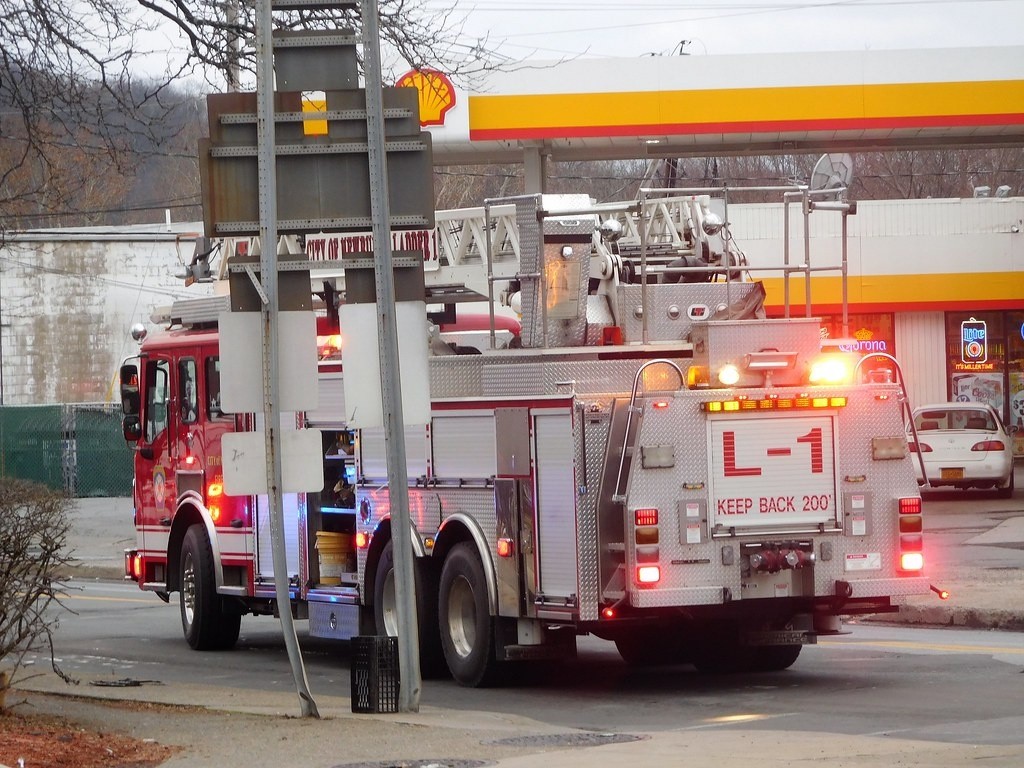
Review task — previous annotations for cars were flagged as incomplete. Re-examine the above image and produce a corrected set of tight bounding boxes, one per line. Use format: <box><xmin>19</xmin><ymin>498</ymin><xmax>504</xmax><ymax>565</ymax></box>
<box><xmin>913</xmin><ymin>401</ymin><xmax>1016</xmax><ymax>491</ymax></box>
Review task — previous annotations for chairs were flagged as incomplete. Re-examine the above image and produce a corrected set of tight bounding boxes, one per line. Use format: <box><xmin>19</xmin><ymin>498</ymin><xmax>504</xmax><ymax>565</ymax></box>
<box><xmin>921</xmin><ymin>422</ymin><xmax>938</xmax><ymax>430</ymax></box>
<box><xmin>966</xmin><ymin>418</ymin><xmax>987</xmax><ymax>430</ymax></box>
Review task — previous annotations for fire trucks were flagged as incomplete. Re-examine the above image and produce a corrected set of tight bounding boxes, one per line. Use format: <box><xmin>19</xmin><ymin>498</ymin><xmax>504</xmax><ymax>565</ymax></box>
<box><xmin>113</xmin><ymin>193</ymin><xmax>926</xmax><ymax>687</ymax></box>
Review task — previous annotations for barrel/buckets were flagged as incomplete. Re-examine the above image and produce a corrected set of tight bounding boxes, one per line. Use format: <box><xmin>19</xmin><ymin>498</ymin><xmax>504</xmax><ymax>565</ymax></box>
<box><xmin>316</xmin><ymin>531</ymin><xmax>349</xmax><ymax>542</ymax></box>
<box><xmin>317</xmin><ymin>542</ymin><xmax>348</xmax><ymax>585</ymax></box>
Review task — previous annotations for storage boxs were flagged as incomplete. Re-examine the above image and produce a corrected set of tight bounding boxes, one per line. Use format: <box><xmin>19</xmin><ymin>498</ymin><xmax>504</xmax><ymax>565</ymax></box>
<box><xmin>350</xmin><ymin>637</ymin><xmax>399</xmax><ymax>714</ymax></box>
